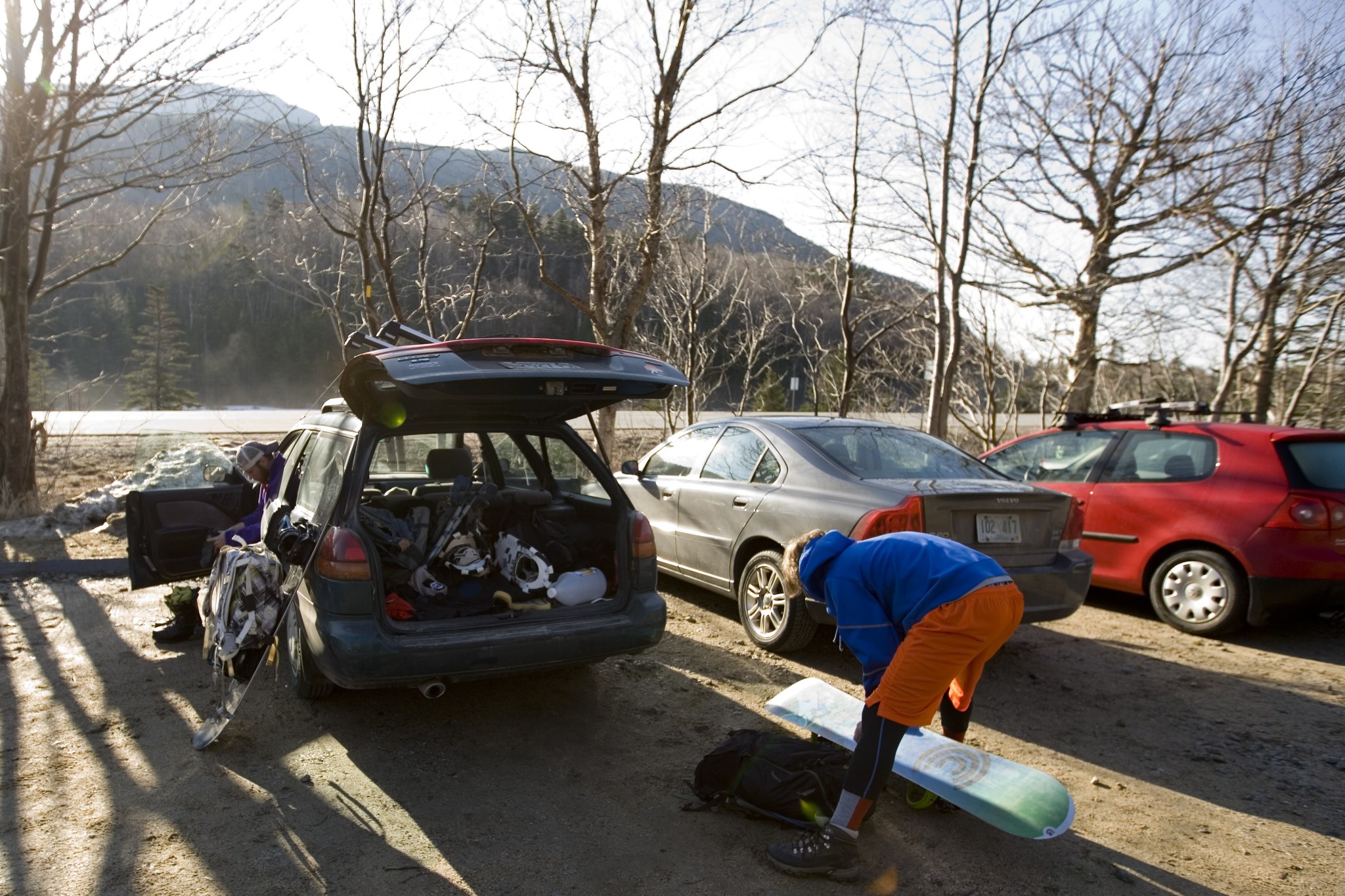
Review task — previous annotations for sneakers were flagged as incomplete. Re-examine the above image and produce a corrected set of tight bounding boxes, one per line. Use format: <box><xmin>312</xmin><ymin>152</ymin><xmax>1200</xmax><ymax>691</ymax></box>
<box><xmin>765</xmin><ymin>822</ymin><xmax>862</xmax><ymax>881</ymax></box>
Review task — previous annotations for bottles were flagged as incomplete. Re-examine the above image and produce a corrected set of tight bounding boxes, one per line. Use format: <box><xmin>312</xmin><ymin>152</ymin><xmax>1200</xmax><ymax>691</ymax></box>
<box><xmin>548</xmin><ymin>567</ymin><xmax>608</xmax><ymax>606</ymax></box>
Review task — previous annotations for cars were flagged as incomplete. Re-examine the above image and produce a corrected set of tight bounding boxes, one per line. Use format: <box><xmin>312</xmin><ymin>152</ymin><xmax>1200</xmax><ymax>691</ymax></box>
<box><xmin>574</xmin><ymin>415</ymin><xmax>1099</xmax><ymax>654</ymax></box>
<box><xmin>124</xmin><ymin>320</ymin><xmax>692</xmax><ymax>721</ymax></box>
<box><xmin>972</xmin><ymin>396</ymin><xmax>1345</xmax><ymax>633</ymax></box>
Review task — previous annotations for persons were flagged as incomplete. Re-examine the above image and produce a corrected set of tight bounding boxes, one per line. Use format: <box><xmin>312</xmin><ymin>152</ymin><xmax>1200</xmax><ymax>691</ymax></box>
<box><xmin>150</xmin><ymin>441</ymin><xmax>299</xmax><ymax>645</ymax></box>
<box><xmin>765</xmin><ymin>529</ymin><xmax>1022</xmax><ymax>882</ymax></box>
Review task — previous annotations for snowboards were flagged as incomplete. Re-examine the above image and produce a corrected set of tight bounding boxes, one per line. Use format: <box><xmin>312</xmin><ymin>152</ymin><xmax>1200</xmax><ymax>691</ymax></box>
<box><xmin>761</xmin><ymin>676</ymin><xmax>1076</xmax><ymax>840</ymax></box>
<box><xmin>191</xmin><ymin>451</ymin><xmax>345</xmax><ymax>752</ymax></box>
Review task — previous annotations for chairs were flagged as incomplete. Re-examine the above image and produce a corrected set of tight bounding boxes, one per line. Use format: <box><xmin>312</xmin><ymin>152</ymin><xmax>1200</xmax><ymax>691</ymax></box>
<box><xmin>1164</xmin><ymin>455</ymin><xmax>1195</xmax><ymax>480</ymax></box>
<box><xmin>411</xmin><ymin>448</ymin><xmax>483</xmax><ymax>495</ymax></box>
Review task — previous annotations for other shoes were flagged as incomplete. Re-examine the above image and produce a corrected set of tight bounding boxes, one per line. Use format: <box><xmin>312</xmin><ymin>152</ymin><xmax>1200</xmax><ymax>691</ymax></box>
<box><xmin>154</xmin><ymin>617</ymin><xmax>175</xmax><ymax>627</ymax></box>
<box><xmin>151</xmin><ymin>619</ymin><xmax>203</xmax><ymax>642</ymax></box>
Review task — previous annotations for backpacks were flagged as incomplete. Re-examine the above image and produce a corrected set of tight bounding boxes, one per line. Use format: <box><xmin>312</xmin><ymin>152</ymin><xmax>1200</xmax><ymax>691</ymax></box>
<box><xmin>202</xmin><ymin>535</ymin><xmax>283</xmax><ymax>714</ymax></box>
<box><xmin>680</xmin><ymin>728</ymin><xmax>876</xmax><ymax>831</ymax></box>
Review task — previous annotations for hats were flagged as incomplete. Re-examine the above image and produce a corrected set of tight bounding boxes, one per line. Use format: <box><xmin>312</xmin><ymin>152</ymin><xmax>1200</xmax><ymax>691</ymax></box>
<box><xmin>236</xmin><ymin>440</ymin><xmax>279</xmax><ymax>472</ymax></box>
